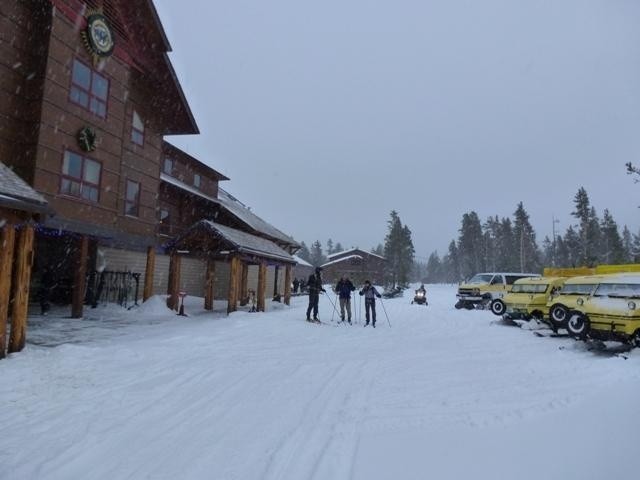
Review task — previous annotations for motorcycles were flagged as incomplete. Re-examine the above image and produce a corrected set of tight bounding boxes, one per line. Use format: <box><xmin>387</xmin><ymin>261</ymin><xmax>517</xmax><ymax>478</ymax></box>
<box><xmin>411</xmin><ymin>289</ymin><xmax>430</xmax><ymax>306</ymax></box>
<box><xmin>382</xmin><ymin>289</ymin><xmax>404</xmax><ymax>300</ymax></box>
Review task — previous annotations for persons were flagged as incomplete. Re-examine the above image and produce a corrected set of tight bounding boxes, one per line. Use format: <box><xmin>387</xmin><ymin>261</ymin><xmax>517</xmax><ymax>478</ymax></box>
<box><xmin>38</xmin><ymin>261</ymin><xmax>59</xmax><ymax>315</ymax></box>
<box><xmin>414</xmin><ymin>284</ymin><xmax>427</xmax><ymax>296</ymax></box>
<box><xmin>293</xmin><ymin>277</ymin><xmax>299</xmax><ymax>293</ymax></box>
<box><xmin>306</xmin><ymin>266</ymin><xmax>327</xmax><ymax>321</ymax></box>
<box><xmin>335</xmin><ymin>272</ymin><xmax>355</xmax><ymax>324</ymax></box>
<box><xmin>359</xmin><ymin>280</ymin><xmax>381</xmax><ymax>326</ymax></box>
<box><xmin>299</xmin><ymin>278</ymin><xmax>305</xmax><ymax>292</ymax></box>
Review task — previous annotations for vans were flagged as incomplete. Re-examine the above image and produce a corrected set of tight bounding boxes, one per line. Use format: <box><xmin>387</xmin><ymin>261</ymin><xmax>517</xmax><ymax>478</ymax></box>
<box><xmin>455</xmin><ymin>263</ymin><xmax>640</xmax><ymax>352</ymax></box>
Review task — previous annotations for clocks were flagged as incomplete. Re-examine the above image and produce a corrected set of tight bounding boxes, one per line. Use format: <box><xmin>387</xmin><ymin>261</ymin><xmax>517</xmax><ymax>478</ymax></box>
<box><xmin>80</xmin><ymin>5</ymin><xmax>115</xmax><ymax>68</ymax></box>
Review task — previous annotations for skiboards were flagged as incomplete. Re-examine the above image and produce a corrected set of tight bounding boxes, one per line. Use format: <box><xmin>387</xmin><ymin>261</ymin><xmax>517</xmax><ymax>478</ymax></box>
<box><xmin>297</xmin><ymin>318</ymin><xmax>376</xmax><ymax>328</ymax></box>
